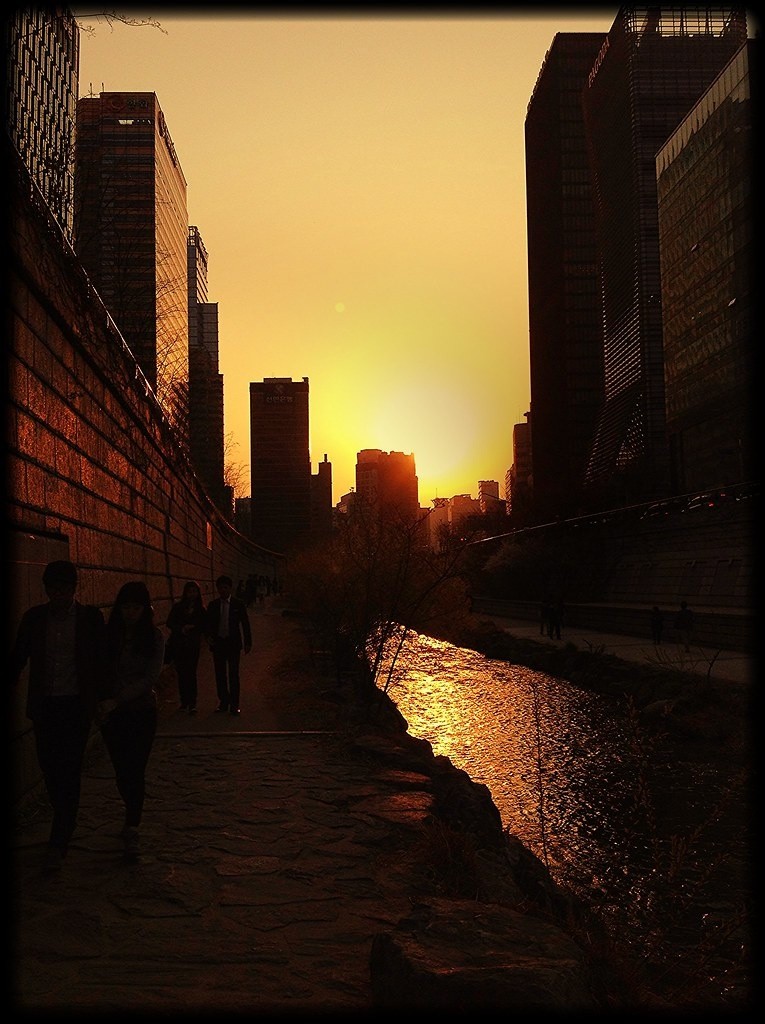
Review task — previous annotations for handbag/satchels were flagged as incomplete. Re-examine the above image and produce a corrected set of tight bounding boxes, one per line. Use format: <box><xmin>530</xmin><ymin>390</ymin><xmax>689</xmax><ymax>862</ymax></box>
<box><xmin>162</xmin><ymin>639</ymin><xmax>175</xmax><ymax>664</ymax></box>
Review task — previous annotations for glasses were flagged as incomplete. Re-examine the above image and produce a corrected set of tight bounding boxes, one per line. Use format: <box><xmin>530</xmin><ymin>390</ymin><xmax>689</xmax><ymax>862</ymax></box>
<box><xmin>46</xmin><ymin>583</ymin><xmax>73</xmax><ymax>595</ymax></box>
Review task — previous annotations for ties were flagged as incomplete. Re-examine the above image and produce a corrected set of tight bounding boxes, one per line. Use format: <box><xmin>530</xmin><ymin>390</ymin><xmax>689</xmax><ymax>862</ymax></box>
<box><xmin>218</xmin><ymin>602</ymin><xmax>226</xmax><ymax>639</ymax></box>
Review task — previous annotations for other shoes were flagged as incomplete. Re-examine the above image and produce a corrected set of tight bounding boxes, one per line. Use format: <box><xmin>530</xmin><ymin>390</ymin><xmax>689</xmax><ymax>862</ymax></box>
<box><xmin>178</xmin><ymin>704</ymin><xmax>186</xmax><ymax>711</ymax></box>
<box><xmin>218</xmin><ymin>700</ymin><xmax>228</xmax><ymax>711</ymax></box>
<box><xmin>188</xmin><ymin>705</ymin><xmax>196</xmax><ymax>713</ymax></box>
<box><xmin>230</xmin><ymin>704</ymin><xmax>240</xmax><ymax>713</ymax></box>
<box><xmin>124</xmin><ymin>827</ymin><xmax>140</xmax><ymax>854</ymax></box>
<box><xmin>120</xmin><ymin>823</ymin><xmax>127</xmax><ymax>838</ymax></box>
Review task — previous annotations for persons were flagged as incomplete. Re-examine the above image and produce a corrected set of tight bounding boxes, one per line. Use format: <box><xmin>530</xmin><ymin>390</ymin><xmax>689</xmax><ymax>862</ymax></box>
<box><xmin>92</xmin><ymin>581</ymin><xmax>165</xmax><ymax>835</ymax></box>
<box><xmin>12</xmin><ymin>559</ymin><xmax>105</xmax><ymax>872</ymax></box>
<box><xmin>236</xmin><ymin>572</ymin><xmax>284</xmax><ymax>607</ymax></box>
<box><xmin>203</xmin><ymin>575</ymin><xmax>252</xmax><ymax>715</ymax></box>
<box><xmin>649</xmin><ymin>606</ymin><xmax>664</xmax><ymax>645</ymax></box>
<box><xmin>538</xmin><ymin>583</ymin><xmax>566</xmax><ymax>641</ymax></box>
<box><xmin>675</xmin><ymin>601</ymin><xmax>696</xmax><ymax>652</ymax></box>
<box><xmin>166</xmin><ymin>580</ymin><xmax>207</xmax><ymax>714</ymax></box>
